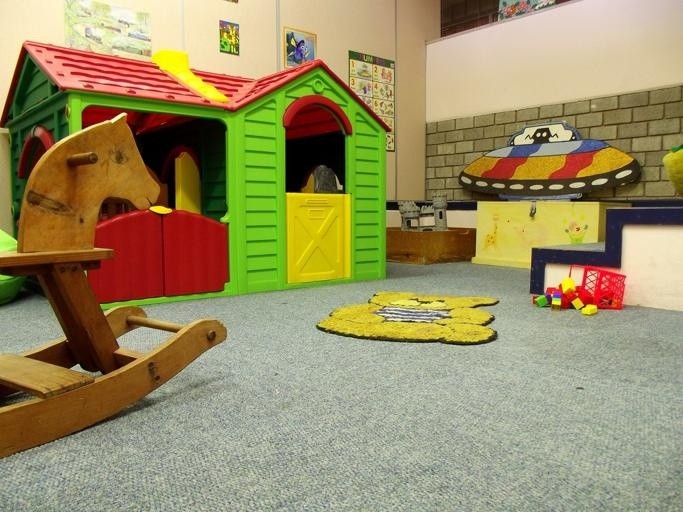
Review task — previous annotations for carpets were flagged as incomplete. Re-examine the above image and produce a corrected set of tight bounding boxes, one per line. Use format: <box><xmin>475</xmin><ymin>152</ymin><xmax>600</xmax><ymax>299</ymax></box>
<box><xmin>316</xmin><ymin>289</ymin><xmax>499</xmax><ymax>345</ymax></box>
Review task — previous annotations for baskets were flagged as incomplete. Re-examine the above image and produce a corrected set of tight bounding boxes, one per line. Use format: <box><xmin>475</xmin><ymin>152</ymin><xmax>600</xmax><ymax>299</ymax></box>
<box><xmin>569</xmin><ymin>265</ymin><xmax>626</xmax><ymax>309</ymax></box>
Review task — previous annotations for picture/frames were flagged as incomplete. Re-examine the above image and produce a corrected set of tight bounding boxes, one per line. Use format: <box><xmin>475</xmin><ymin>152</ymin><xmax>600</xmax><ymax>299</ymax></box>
<box><xmin>283</xmin><ymin>26</ymin><xmax>317</xmax><ymax>71</ymax></box>
